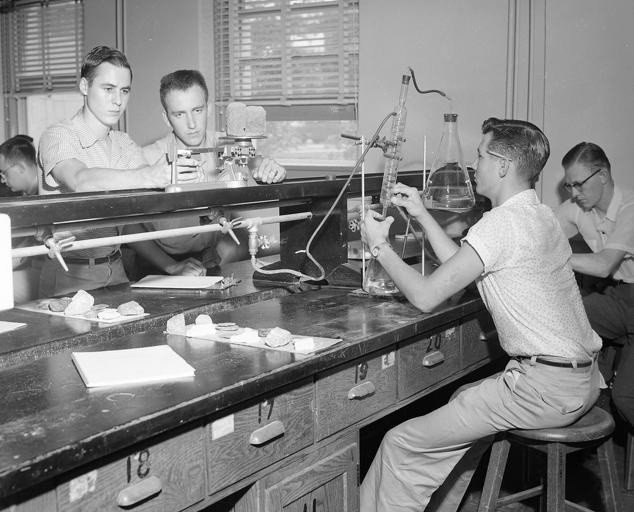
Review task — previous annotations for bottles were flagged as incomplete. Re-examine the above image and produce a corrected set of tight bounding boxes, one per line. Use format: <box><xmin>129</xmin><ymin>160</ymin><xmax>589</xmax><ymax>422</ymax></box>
<box><xmin>422</xmin><ymin>114</ymin><xmax>476</xmax><ymax>213</ymax></box>
<box><xmin>380</xmin><ymin>73</ymin><xmax>411</xmax><ymax>206</ymax></box>
<box><xmin>362</xmin><ymin>233</ymin><xmax>403</xmax><ymax>296</ymax></box>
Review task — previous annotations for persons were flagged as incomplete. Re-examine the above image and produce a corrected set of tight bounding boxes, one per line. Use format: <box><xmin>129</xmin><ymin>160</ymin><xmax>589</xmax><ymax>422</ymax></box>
<box><xmin>553</xmin><ymin>142</ymin><xmax>634</xmax><ymax>427</ymax></box>
<box><xmin>122</xmin><ymin>71</ymin><xmax>288</xmax><ymax>281</ymax></box>
<box><xmin>358</xmin><ymin>118</ymin><xmax>610</xmax><ymax>512</ymax></box>
<box><xmin>0</xmin><ymin>137</ymin><xmax>39</xmax><ymax>198</ymax></box>
<box><xmin>38</xmin><ymin>47</ymin><xmax>201</xmax><ymax>297</ymax></box>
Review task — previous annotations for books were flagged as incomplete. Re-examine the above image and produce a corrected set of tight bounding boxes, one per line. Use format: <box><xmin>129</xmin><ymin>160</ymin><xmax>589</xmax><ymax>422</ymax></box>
<box><xmin>130</xmin><ymin>275</ymin><xmax>224</xmax><ymax>288</ymax></box>
<box><xmin>71</xmin><ymin>345</ymin><xmax>196</xmax><ymax>388</ymax></box>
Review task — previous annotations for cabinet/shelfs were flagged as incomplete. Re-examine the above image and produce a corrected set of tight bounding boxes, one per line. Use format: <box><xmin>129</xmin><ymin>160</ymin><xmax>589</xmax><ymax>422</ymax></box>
<box><xmin>0</xmin><ymin>307</ymin><xmax>507</xmax><ymax>512</ymax></box>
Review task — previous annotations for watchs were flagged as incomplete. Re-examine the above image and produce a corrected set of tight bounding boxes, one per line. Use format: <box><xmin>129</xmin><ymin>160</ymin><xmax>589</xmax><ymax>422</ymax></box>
<box><xmin>371</xmin><ymin>240</ymin><xmax>388</xmax><ymax>260</ymax></box>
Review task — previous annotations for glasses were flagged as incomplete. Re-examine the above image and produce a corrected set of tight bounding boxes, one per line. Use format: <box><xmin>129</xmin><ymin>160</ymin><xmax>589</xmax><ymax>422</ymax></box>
<box><xmin>563</xmin><ymin>169</ymin><xmax>601</xmax><ymax>192</ymax></box>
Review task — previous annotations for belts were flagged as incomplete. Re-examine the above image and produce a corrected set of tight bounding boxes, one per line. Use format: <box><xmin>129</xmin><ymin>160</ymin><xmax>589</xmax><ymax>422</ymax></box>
<box><xmin>63</xmin><ymin>252</ymin><xmax>120</xmax><ymax>264</ymax></box>
<box><xmin>516</xmin><ymin>354</ymin><xmax>596</xmax><ymax>366</ymax></box>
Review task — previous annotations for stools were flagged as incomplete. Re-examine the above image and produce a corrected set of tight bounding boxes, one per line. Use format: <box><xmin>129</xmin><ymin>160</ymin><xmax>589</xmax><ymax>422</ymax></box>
<box><xmin>604</xmin><ymin>340</ymin><xmax>633</xmax><ymax>492</ymax></box>
<box><xmin>474</xmin><ymin>404</ymin><xmax>617</xmax><ymax>511</ymax></box>
